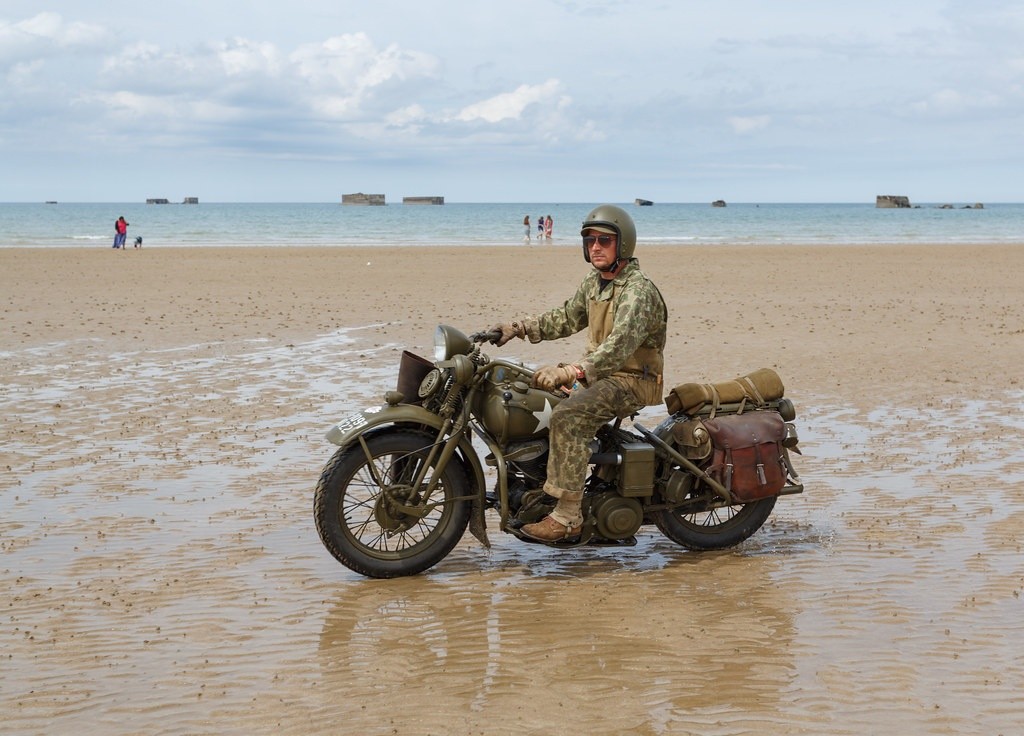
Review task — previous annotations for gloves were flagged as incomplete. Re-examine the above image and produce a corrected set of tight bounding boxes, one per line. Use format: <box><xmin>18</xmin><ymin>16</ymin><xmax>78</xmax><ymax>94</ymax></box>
<box><xmin>531</xmin><ymin>362</ymin><xmax>577</xmax><ymax>393</ymax></box>
<box><xmin>486</xmin><ymin>320</ymin><xmax>526</xmax><ymax>347</ymax></box>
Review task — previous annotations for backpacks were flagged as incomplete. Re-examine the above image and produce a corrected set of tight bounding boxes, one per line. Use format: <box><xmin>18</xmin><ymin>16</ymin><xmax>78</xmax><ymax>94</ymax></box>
<box><xmin>700</xmin><ymin>407</ymin><xmax>799</xmax><ymax>504</ymax></box>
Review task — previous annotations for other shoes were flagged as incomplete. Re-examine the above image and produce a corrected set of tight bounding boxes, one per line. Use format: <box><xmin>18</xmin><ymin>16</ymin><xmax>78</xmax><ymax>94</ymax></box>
<box><xmin>519</xmin><ymin>515</ymin><xmax>582</xmax><ymax>543</ymax></box>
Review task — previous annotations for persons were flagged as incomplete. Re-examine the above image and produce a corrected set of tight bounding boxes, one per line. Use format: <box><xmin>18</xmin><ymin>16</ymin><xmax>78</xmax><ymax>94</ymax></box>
<box><xmin>536</xmin><ymin>214</ymin><xmax>554</xmax><ymax>240</ymax></box>
<box><xmin>111</xmin><ymin>216</ymin><xmax>129</xmax><ymax>249</ymax></box>
<box><xmin>523</xmin><ymin>215</ymin><xmax>530</xmax><ymax>240</ymax></box>
<box><xmin>486</xmin><ymin>204</ymin><xmax>667</xmax><ymax>545</ymax></box>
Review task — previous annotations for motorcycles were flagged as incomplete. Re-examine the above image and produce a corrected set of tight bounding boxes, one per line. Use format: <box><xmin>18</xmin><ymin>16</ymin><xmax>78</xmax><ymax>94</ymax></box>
<box><xmin>309</xmin><ymin>320</ymin><xmax>806</xmax><ymax>580</ymax></box>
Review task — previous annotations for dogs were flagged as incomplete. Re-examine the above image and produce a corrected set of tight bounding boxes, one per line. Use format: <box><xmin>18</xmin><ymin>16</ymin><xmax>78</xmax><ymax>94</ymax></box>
<box><xmin>134</xmin><ymin>236</ymin><xmax>142</xmax><ymax>247</ymax></box>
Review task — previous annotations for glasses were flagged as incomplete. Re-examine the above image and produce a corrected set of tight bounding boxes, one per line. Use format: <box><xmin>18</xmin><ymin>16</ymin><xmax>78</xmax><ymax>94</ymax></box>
<box><xmin>583</xmin><ymin>234</ymin><xmax>617</xmax><ymax>249</ymax></box>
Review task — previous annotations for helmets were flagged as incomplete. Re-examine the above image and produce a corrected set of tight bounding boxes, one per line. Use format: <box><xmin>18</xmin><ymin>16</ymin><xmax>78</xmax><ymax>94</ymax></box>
<box><xmin>580</xmin><ymin>204</ymin><xmax>637</xmax><ymax>264</ymax></box>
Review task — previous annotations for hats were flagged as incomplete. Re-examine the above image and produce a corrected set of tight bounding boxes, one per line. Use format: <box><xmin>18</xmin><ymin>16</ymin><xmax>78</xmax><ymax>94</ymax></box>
<box><xmin>580</xmin><ymin>225</ymin><xmax>619</xmax><ymax>237</ymax></box>
